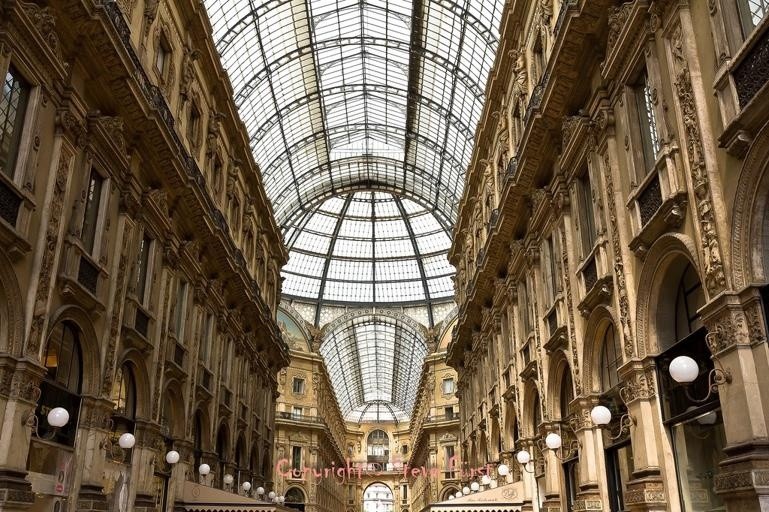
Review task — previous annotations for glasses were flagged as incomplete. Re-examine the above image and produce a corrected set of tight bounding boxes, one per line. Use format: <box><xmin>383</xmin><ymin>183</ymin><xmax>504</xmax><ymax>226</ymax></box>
<box><xmin>591</xmin><ymin>385</ymin><xmax>637</xmax><ymax>443</ymax></box>
<box><xmin>671</xmin><ymin>327</ymin><xmax>738</xmax><ymax>411</ymax></box>
<box><xmin>97</xmin><ymin>413</ymin><xmax>138</xmax><ymax>466</ymax></box>
<box><xmin>545</xmin><ymin>413</ymin><xmax>584</xmax><ymax>461</ymax></box>
<box><xmin>516</xmin><ymin>448</ymin><xmax>544</xmax><ymax>478</ymax></box>
<box><xmin>21</xmin><ymin>381</ymin><xmax>69</xmax><ymax>442</ymax></box>
<box><xmin>497</xmin><ymin>462</ymin><xmax>515</xmax><ymax>483</ymax></box>
<box><xmin>449</xmin><ymin>474</ymin><xmax>497</xmax><ymax>500</ymax></box>
<box><xmin>149</xmin><ymin>438</ymin><xmax>180</xmax><ymax>475</ymax></box>
<box><xmin>221</xmin><ymin>474</ymin><xmax>287</xmax><ymax>506</ymax></box>
<box><xmin>184</xmin><ymin>454</ymin><xmax>211</xmax><ymax>488</ymax></box>
